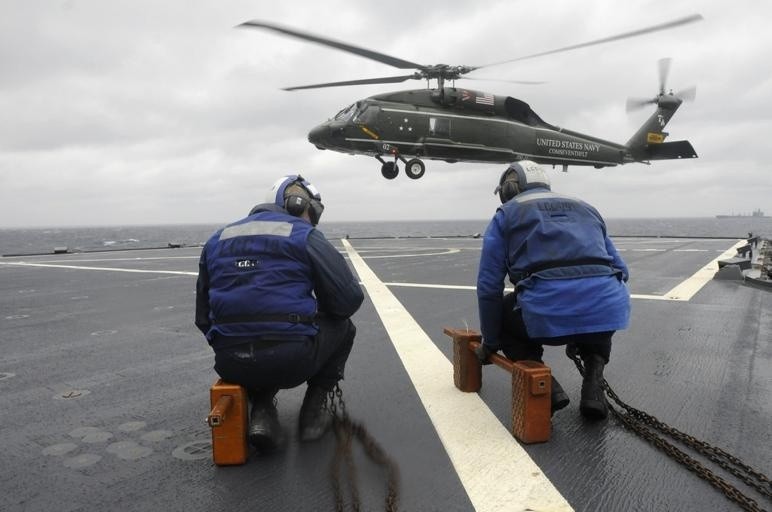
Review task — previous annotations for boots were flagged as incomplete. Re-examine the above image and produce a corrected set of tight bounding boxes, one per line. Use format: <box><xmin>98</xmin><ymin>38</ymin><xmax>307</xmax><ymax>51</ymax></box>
<box><xmin>298</xmin><ymin>384</ymin><xmax>333</xmax><ymax>442</ymax></box>
<box><xmin>248</xmin><ymin>393</ymin><xmax>280</xmax><ymax>441</ymax></box>
<box><xmin>579</xmin><ymin>358</ymin><xmax>608</xmax><ymax>416</ymax></box>
<box><xmin>551</xmin><ymin>376</ymin><xmax>570</xmax><ymax>420</ymax></box>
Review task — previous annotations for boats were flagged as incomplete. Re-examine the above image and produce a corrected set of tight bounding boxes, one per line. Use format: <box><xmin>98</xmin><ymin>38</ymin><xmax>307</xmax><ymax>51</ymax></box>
<box><xmin>716</xmin><ymin>209</ymin><xmax>771</xmax><ymax>218</ymax></box>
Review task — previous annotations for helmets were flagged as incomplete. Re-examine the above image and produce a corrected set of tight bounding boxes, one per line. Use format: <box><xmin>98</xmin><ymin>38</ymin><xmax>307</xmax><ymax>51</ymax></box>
<box><xmin>493</xmin><ymin>158</ymin><xmax>551</xmax><ymax>202</ymax></box>
<box><xmin>264</xmin><ymin>174</ymin><xmax>324</xmax><ymax>224</ymax></box>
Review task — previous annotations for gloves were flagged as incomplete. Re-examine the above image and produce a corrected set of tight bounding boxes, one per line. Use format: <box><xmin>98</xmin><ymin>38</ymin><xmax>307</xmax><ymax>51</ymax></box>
<box><xmin>477</xmin><ymin>337</ymin><xmax>500</xmax><ymax>366</ymax></box>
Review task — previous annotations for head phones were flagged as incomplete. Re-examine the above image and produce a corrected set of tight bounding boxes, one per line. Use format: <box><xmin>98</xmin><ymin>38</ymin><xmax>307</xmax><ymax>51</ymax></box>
<box><xmin>492</xmin><ymin>168</ymin><xmax>520</xmax><ymax>202</ymax></box>
<box><xmin>287</xmin><ymin>186</ymin><xmax>324</xmax><ymax>223</ymax></box>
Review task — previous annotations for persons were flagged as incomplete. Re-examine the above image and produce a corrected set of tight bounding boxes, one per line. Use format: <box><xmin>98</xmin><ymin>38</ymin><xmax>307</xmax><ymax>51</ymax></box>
<box><xmin>476</xmin><ymin>158</ymin><xmax>631</xmax><ymax>421</ymax></box>
<box><xmin>194</xmin><ymin>173</ymin><xmax>365</xmax><ymax>446</ymax></box>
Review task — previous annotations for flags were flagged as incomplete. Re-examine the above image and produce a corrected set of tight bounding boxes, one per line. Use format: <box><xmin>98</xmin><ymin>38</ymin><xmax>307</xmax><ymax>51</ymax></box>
<box><xmin>475</xmin><ymin>89</ymin><xmax>494</xmax><ymax>107</ymax></box>
<box><xmin>461</xmin><ymin>90</ymin><xmax>474</xmax><ymax>102</ymax></box>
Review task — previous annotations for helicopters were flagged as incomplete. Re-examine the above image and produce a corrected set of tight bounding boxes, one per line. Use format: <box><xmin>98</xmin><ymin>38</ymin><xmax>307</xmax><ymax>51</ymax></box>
<box><xmin>238</xmin><ymin>12</ymin><xmax>706</xmax><ymax>179</ymax></box>
